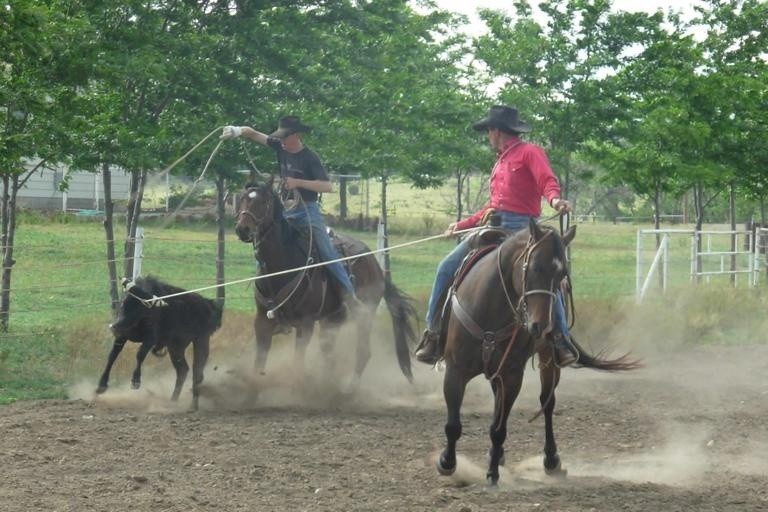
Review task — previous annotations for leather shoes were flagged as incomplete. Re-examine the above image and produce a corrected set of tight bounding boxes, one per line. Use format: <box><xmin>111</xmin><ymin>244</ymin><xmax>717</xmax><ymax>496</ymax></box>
<box><xmin>416</xmin><ymin>340</ymin><xmax>436</xmax><ymax>361</ymax></box>
<box><xmin>559</xmin><ymin>347</ymin><xmax>576</xmax><ymax>365</ymax></box>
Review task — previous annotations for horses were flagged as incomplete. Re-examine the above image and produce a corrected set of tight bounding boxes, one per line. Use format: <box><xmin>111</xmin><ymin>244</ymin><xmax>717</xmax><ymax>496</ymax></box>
<box><xmin>233</xmin><ymin>167</ymin><xmax>420</xmax><ymax>392</ymax></box>
<box><xmin>435</xmin><ymin>217</ymin><xmax>640</xmax><ymax>488</ymax></box>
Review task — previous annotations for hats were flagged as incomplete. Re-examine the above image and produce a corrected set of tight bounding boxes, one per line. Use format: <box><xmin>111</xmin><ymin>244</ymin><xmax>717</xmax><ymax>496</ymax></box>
<box><xmin>271</xmin><ymin>117</ymin><xmax>308</xmax><ymax>138</ymax></box>
<box><xmin>472</xmin><ymin>106</ymin><xmax>531</xmax><ymax>133</ymax></box>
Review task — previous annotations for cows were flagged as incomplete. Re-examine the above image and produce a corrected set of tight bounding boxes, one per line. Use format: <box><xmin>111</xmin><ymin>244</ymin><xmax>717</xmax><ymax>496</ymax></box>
<box><xmin>95</xmin><ymin>273</ymin><xmax>223</xmax><ymax>413</ymax></box>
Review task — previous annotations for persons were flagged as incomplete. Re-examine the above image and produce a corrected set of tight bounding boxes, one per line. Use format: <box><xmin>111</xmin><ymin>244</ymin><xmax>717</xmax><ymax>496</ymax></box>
<box><xmin>415</xmin><ymin>105</ymin><xmax>578</xmax><ymax>367</ymax></box>
<box><xmin>218</xmin><ymin>116</ymin><xmax>358</xmax><ymax>306</ymax></box>
